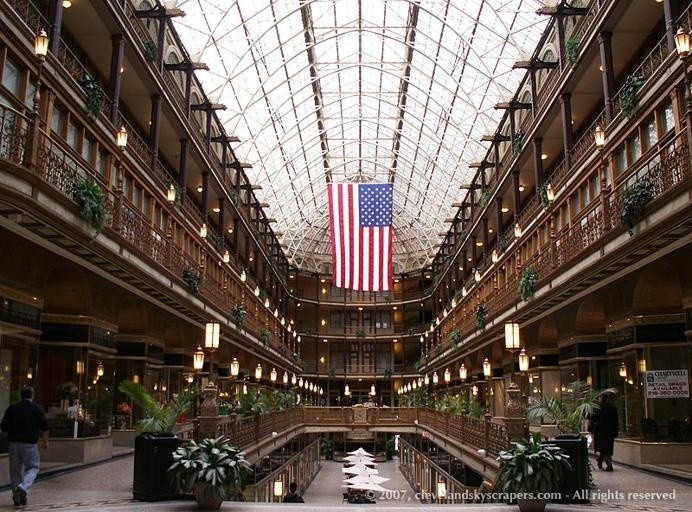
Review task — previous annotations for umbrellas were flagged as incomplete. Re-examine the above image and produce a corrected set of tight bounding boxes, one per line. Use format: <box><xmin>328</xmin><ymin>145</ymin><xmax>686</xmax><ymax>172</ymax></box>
<box><xmin>339</xmin><ymin>446</ymin><xmax>394</xmax><ymax>498</ymax></box>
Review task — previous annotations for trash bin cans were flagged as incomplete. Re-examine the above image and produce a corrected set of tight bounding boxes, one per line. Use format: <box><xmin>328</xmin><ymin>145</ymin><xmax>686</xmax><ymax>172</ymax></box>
<box><xmin>386</xmin><ymin>451</ymin><xmax>392</xmax><ymax>460</ymax></box>
<box><xmin>325</xmin><ymin>449</ymin><xmax>332</xmax><ymax>460</ymax></box>
<box><xmin>133</xmin><ymin>432</ymin><xmax>181</xmax><ymax>502</ymax></box>
<box><xmin>541</xmin><ymin>433</ymin><xmax>590</xmax><ymax>504</ymax></box>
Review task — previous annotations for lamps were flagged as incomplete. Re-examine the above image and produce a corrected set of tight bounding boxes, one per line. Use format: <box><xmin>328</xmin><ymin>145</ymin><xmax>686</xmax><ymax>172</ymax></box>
<box><xmin>675</xmin><ymin>23</ymin><xmax>689</xmax><ymax>63</ymax></box>
<box><xmin>394</xmin><ymin>303</ymin><xmax>533</xmax><ymax>394</ymax></box>
<box><xmin>116</xmin><ymin>124</ymin><xmax>128</xmax><ymax>150</ymax></box>
<box><xmin>165</xmin><ymin>182</ymin><xmax>271</xmax><ymax>309</ymax></box>
<box><xmin>35</xmin><ymin>25</ymin><xmax>50</xmax><ymax>63</ymax></box>
<box><xmin>448</xmin><ymin>180</ymin><xmax>556</xmax><ymax>308</ymax></box>
<box><xmin>191</xmin><ymin>311</ymin><xmax>327</xmax><ymax>395</ymax></box>
<box><xmin>595</xmin><ymin>123</ymin><xmax>604</xmax><ymax>152</ymax></box>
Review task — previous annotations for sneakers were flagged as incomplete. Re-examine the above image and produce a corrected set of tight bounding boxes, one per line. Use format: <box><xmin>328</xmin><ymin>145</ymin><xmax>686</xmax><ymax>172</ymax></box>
<box><xmin>12</xmin><ymin>486</ymin><xmax>28</xmax><ymax>506</ymax></box>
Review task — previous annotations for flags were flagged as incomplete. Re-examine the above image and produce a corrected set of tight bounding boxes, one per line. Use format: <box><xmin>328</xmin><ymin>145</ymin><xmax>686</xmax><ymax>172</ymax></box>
<box><xmin>325</xmin><ymin>181</ymin><xmax>395</xmax><ymax>292</ymax></box>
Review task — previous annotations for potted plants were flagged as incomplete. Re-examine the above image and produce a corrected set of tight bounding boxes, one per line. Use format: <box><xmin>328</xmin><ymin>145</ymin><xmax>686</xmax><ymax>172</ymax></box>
<box><xmin>116</xmin><ymin>372</ymin><xmax>255</xmax><ymax>512</ymax></box>
<box><xmin>488</xmin><ymin>386</ymin><xmax>618</xmax><ymax>512</ymax></box>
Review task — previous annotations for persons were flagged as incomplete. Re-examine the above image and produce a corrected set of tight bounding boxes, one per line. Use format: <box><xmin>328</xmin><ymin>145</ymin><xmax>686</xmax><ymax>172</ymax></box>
<box><xmin>0</xmin><ymin>386</ymin><xmax>49</xmax><ymax>507</ymax></box>
<box><xmin>283</xmin><ymin>482</ymin><xmax>306</xmax><ymax>502</ymax></box>
<box><xmin>588</xmin><ymin>393</ymin><xmax>620</xmax><ymax>474</ymax></box>
